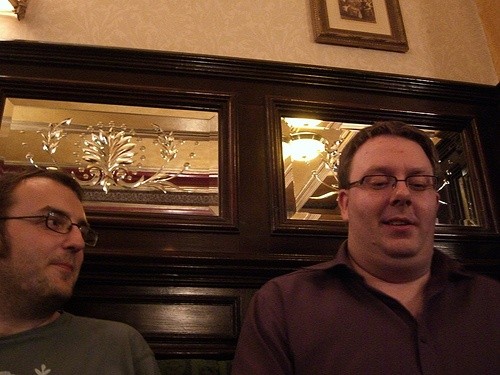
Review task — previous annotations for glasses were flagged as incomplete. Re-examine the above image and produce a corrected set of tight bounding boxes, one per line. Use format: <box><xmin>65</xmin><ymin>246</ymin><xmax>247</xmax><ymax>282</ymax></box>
<box><xmin>0</xmin><ymin>211</ymin><xmax>98</xmax><ymax>248</ymax></box>
<box><xmin>342</xmin><ymin>175</ymin><xmax>439</xmax><ymax>193</ymax></box>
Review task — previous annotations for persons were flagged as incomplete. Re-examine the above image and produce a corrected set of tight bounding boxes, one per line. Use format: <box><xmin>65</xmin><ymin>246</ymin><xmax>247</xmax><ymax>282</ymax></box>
<box><xmin>229</xmin><ymin>118</ymin><xmax>500</xmax><ymax>375</ymax></box>
<box><xmin>0</xmin><ymin>166</ymin><xmax>163</xmax><ymax>375</ymax></box>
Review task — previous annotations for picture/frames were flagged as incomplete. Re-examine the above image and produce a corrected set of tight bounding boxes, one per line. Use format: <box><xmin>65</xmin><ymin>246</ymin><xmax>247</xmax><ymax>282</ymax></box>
<box><xmin>309</xmin><ymin>0</ymin><xmax>409</xmax><ymax>53</ymax></box>
